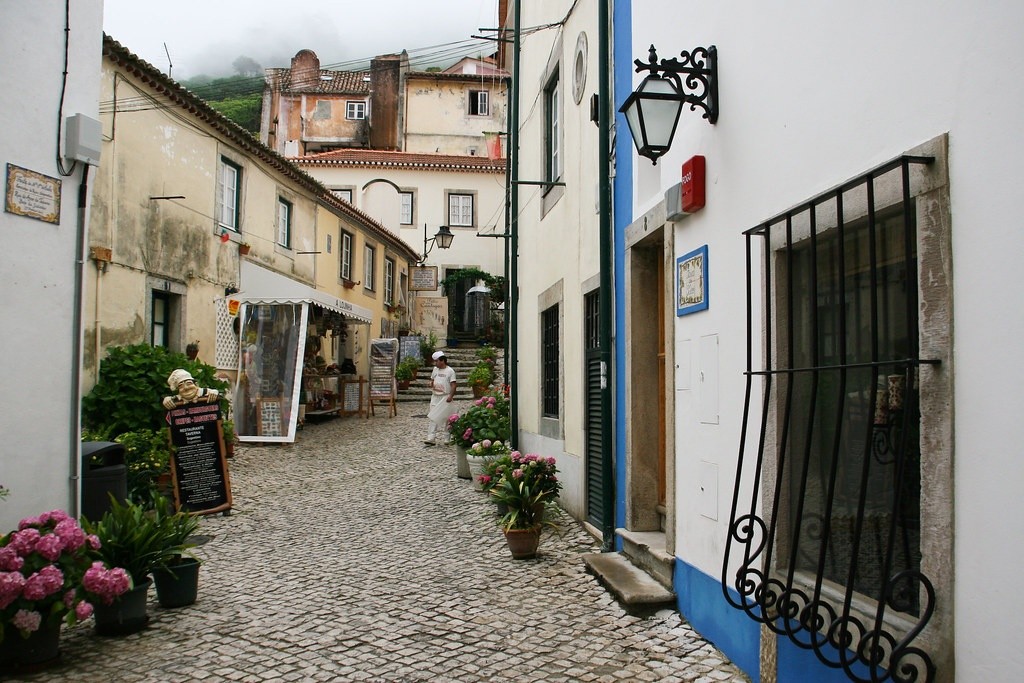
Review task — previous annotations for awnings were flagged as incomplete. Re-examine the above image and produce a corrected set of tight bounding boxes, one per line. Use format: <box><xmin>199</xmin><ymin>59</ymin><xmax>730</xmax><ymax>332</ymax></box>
<box><xmin>227</xmin><ymin>260</ymin><xmax>374</xmax><ymax>326</ymax></box>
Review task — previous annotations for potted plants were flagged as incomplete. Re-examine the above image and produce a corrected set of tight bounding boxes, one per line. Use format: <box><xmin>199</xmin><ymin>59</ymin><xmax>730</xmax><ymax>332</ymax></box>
<box><xmin>399</xmin><ymin>322</ymin><xmax>412</xmax><ymax>336</ymax></box>
<box><xmin>489</xmin><ymin>464</ymin><xmax>563</xmax><ymax>559</ymax></box>
<box><xmin>223</xmin><ymin>418</ymin><xmax>236</xmax><ymax>458</ymax></box>
<box><xmin>388</xmin><ymin>298</ymin><xmax>396</xmax><ymax>313</ymax></box>
<box><xmin>465</xmin><ymin>359</ymin><xmax>494</xmax><ymax>397</ymax></box>
<box><xmin>396</xmin><ymin>359</ymin><xmax>414</xmax><ymax>390</ymax></box>
<box><xmin>77</xmin><ymin>490</ymin><xmax>191</xmax><ymax>636</ymax></box>
<box><xmin>474</xmin><ymin>341</ymin><xmax>500</xmax><ymax>365</ymax></box>
<box><xmin>149</xmin><ymin>480</ymin><xmax>202</xmax><ymax>609</ymax></box>
<box><xmin>420</xmin><ymin>330</ymin><xmax>438</xmax><ymax>366</ymax></box>
<box><xmin>404</xmin><ymin>354</ymin><xmax>419</xmax><ymax>381</ymax></box>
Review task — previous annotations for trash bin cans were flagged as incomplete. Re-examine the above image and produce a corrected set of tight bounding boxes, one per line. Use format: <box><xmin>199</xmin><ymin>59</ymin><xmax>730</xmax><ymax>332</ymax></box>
<box><xmin>81</xmin><ymin>440</ymin><xmax>129</xmax><ymax>522</ymax></box>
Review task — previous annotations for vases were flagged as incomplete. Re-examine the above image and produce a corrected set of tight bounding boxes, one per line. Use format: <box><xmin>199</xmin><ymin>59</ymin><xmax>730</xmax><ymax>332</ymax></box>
<box><xmin>456</xmin><ymin>437</ymin><xmax>471</xmax><ymax>479</ymax></box>
<box><xmin>343</xmin><ymin>279</ymin><xmax>356</xmax><ymax>289</ymax></box>
<box><xmin>465</xmin><ymin>453</ymin><xmax>502</xmax><ymax>493</ymax></box>
<box><xmin>497</xmin><ymin>501</ymin><xmax>508</xmax><ymax>515</ymax></box>
<box><xmin>507</xmin><ymin>502</ymin><xmax>545</xmax><ymax>524</ymax></box>
<box><xmin>155</xmin><ymin>471</ymin><xmax>172</xmax><ymax>490</ymax></box>
<box><xmin>239</xmin><ymin>244</ymin><xmax>251</xmax><ymax>255</ymax></box>
<box><xmin>490</xmin><ymin>365</ymin><xmax>494</xmax><ymax>370</ymax></box>
<box><xmin>3</xmin><ymin>607</ymin><xmax>64</xmax><ymax>666</ymax></box>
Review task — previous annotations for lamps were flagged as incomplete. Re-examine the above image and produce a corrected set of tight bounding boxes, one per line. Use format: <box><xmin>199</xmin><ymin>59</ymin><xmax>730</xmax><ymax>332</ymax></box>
<box><xmin>617</xmin><ymin>44</ymin><xmax>719</xmax><ymax>168</ymax></box>
<box><xmin>417</xmin><ymin>221</ymin><xmax>455</xmax><ymax>263</ymax></box>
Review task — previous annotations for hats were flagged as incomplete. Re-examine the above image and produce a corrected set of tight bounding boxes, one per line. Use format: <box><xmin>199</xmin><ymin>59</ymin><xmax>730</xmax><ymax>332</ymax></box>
<box><xmin>432</xmin><ymin>350</ymin><xmax>444</xmax><ymax>360</ymax></box>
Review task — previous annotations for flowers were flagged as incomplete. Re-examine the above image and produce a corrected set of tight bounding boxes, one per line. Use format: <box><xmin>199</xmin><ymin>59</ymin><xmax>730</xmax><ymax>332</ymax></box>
<box><xmin>0</xmin><ymin>493</ymin><xmax>132</xmax><ymax>637</ymax></box>
<box><xmin>477</xmin><ymin>358</ymin><xmax>495</xmax><ymax>366</ymax></box>
<box><xmin>445</xmin><ymin>383</ymin><xmax>563</xmax><ymax>500</ymax></box>
<box><xmin>240</xmin><ymin>239</ymin><xmax>248</xmax><ymax>246</ymax></box>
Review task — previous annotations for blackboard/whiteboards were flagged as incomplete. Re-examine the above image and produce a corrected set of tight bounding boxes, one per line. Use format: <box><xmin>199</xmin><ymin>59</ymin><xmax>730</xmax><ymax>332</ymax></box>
<box><xmin>167</xmin><ymin>397</ymin><xmax>233</xmax><ymax>516</ymax></box>
<box><xmin>341</xmin><ymin>379</ymin><xmax>363</xmax><ymax>414</ymax></box>
<box><xmin>368</xmin><ymin>339</ymin><xmax>397</xmax><ymax>399</ymax></box>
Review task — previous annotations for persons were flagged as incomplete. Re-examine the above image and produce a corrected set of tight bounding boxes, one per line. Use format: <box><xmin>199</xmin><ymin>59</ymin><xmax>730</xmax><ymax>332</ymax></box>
<box><xmin>163</xmin><ymin>369</ymin><xmax>219</xmax><ymax>410</ymax></box>
<box><xmin>424</xmin><ymin>350</ymin><xmax>456</xmax><ymax>446</ymax></box>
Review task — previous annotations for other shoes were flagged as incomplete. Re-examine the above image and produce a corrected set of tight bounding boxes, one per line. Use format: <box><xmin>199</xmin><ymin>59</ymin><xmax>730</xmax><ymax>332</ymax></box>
<box><xmin>424</xmin><ymin>439</ymin><xmax>436</xmax><ymax>445</ymax></box>
<box><xmin>444</xmin><ymin>438</ymin><xmax>450</xmax><ymax>445</ymax></box>
